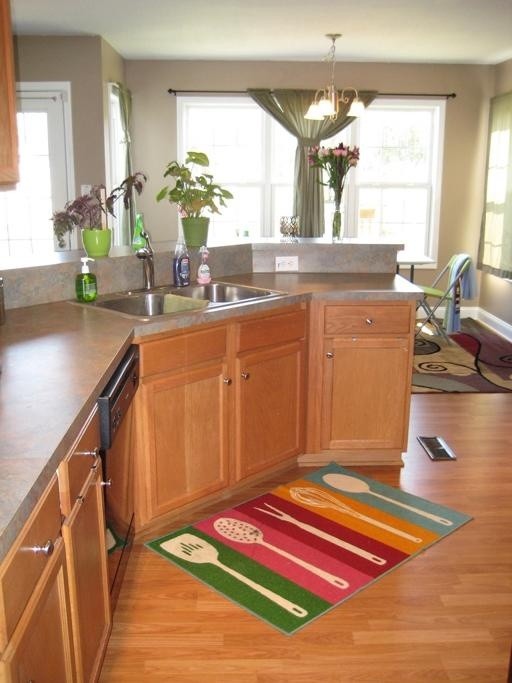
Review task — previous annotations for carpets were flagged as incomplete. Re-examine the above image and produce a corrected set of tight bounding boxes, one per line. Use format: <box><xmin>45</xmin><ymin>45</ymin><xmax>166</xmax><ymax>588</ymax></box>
<box><xmin>140</xmin><ymin>460</ymin><xmax>474</xmax><ymax>634</ymax></box>
<box><xmin>411</xmin><ymin>316</ymin><xmax>512</xmax><ymax>394</ymax></box>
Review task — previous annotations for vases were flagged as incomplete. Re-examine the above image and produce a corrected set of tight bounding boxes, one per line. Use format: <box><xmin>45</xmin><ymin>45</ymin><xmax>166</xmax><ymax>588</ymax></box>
<box><xmin>334</xmin><ymin>188</ymin><xmax>343</xmax><ymax>238</ymax></box>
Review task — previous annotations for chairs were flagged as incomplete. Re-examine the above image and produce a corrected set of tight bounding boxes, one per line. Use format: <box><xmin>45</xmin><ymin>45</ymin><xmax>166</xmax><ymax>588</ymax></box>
<box><xmin>414</xmin><ymin>254</ymin><xmax>472</xmax><ymax>346</ymax></box>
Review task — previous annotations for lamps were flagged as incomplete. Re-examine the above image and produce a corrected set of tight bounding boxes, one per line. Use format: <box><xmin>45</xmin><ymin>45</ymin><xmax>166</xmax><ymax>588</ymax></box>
<box><xmin>304</xmin><ymin>32</ymin><xmax>367</xmax><ymax>121</ymax></box>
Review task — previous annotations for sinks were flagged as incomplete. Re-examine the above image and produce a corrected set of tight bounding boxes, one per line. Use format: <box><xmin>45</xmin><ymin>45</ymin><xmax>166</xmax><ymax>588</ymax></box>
<box><xmin>167</xmin><ymin>282</ymin><xmax>288</xmax><ymax>306</ymax></box>
<box><xmin>68</xmin><ymin>293</ymin><xmax>209</xmax><ymax>321</ymax></box>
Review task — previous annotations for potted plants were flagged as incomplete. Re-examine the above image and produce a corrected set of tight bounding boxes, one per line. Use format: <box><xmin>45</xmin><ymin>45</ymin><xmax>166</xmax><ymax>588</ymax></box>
<box><xmin>155</xmin><ymin>152</ymin><xmax>233</xmax><ymax>248</ymax></box>
<box><xmin>47</xmin><ymin>170</ymin><xmax>147</xmax><ymax>258</ymax></box>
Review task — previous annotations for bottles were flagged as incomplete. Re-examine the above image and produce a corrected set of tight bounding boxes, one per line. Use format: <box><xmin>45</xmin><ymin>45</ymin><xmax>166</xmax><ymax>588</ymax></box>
<box><xmin>196</xmin><ymin>245</ymin><xmax>212</xmax><ymax>281</ymax></box>
<box><xmin>132</xmin><ymin>213</ymin><xmax>147</xmax><ymax>251</ymax></box>
<box><xmin>174</xmin><ymin>231</ymin><xmax>192</xmax><ymax>288</ymax></box>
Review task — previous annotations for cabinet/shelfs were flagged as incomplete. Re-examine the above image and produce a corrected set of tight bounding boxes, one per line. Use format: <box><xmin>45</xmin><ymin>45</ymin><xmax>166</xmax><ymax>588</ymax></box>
<box><xmin>129</xmin><ymin>301</ymin><xmax>310</xmax><ymax>539</ymax></box>
<box><xmin>0</xmin><ymin>402</ymin><xmax>113</xmax><ymax>683</ymax></box>
<box><xmin>0</xmin><ymin>0</ymin><xmax>20</xmax><ymax>192</ymax></box>
<box><xmin>101</xmin><ymin>343</ymin><xmax>134</xmax><ymax>614</ymax></box>
<box><xmin>310</xmin><ymin>295</ymin><xmax>413</xmax><ymax>470</ymax></box>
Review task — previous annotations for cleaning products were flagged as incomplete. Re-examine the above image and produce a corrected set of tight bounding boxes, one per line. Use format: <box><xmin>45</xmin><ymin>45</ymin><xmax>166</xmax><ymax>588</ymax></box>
<box><xmin>173</xmin><ymin>230</ymin><xmax>190</xmax><ymax>287</ymax></box>
<box><xmin>133</xmin><ymin>212</ymin><xmax>146</xmax><ymax>253</ymax></box>
<box><xmin>196</xmin><ymin>246</ymin><xmax>212</xmax><ymax>285</ymax></box>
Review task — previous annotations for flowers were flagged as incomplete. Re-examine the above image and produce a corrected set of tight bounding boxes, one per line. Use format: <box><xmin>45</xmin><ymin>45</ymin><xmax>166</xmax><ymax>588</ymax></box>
<box><xmin>307</xmin><ymin>142</ymin><xmax>359</xmax><ymax>239</ymax></box>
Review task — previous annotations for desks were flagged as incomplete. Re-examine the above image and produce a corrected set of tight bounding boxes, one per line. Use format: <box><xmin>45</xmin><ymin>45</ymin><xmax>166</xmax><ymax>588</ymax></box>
<box><xmin>396</xmin><ymin>253</ymin><xmax>435</xmax><ymax>282</ymax></box>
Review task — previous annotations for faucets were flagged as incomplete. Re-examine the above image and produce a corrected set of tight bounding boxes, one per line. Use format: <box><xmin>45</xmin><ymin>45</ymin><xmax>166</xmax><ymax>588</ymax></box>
<box><xmin>134</xmin><ymin>232</ymin><xmax>154</xmax><ymax>289</ymax></box>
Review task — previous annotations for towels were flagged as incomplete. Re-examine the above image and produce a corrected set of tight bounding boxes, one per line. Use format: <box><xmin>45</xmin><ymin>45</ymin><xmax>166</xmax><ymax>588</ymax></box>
<box><xmin>442</xmin><ymin>253</ymin><xmax>475</xmax><ymax>334</ymax></box>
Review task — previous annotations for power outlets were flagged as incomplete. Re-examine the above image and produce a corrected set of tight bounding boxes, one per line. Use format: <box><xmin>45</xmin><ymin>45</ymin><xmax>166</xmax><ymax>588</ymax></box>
<box><xmin>275</xmin><ymin>256</ymin><xmax>298</xmax><ymax>271</ymax></box>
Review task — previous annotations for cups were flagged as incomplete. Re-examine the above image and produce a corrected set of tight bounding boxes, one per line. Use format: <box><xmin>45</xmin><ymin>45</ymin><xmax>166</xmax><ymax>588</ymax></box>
<box><xmin>280</xmin><ymin>215</ymin><xmax>301</xmax><ymax>243</ymax></box>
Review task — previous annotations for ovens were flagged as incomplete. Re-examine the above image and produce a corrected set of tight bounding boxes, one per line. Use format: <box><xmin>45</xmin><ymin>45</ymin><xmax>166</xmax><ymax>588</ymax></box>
<box><xmin>96</xmin><ymin>339</ymin><xmax>142</xmax><ymax>618</ymax></box>
